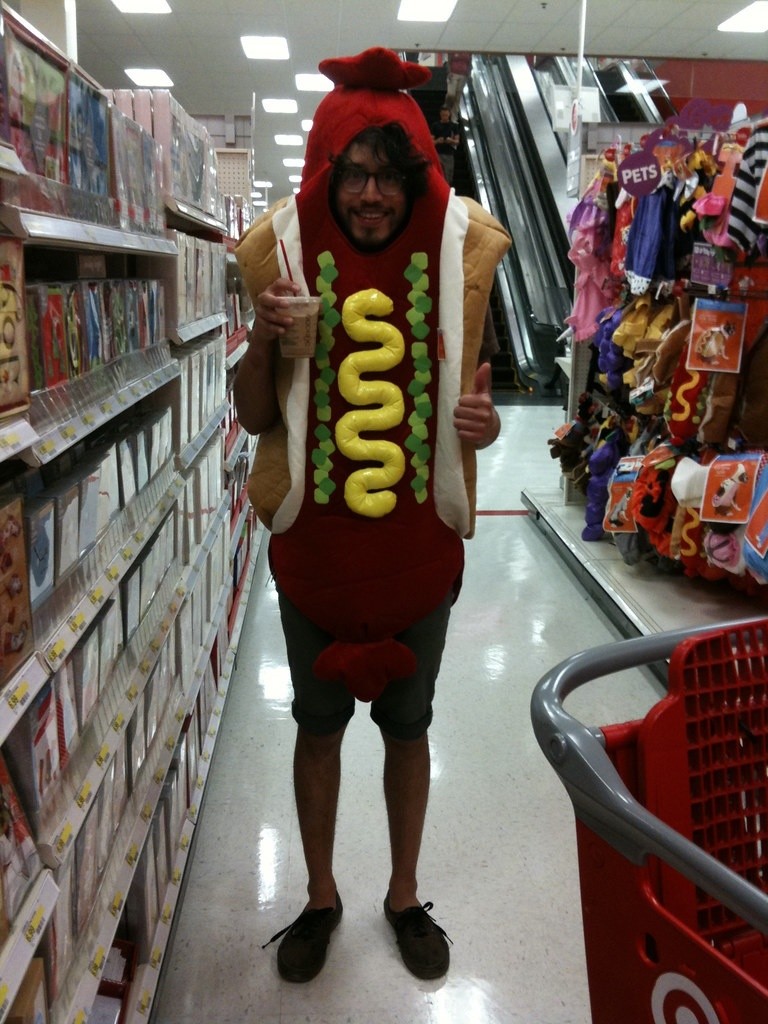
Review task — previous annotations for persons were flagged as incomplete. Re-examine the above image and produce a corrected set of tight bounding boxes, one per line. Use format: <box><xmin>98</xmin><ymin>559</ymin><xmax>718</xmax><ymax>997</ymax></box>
<box><xmin>231</xmin><ymin>47</ymin><xmax>512</xmax><ymax>984</ymax></box>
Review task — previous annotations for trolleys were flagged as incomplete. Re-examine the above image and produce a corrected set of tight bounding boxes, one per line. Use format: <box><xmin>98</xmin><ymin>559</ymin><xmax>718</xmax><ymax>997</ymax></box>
<box><xmin>531</xmin><ymin>612</ymin><xmax>768</xmax><ymax>1024</ymax></box>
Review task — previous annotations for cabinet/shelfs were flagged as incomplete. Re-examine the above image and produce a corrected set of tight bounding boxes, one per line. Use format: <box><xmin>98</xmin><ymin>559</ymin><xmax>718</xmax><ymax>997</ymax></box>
<box><xmin>164</xmin><ymin>196</ymin><xmax>264</xmax><ymax>848</ymax></box>
<box><xmin>0</xmin><ymin>0</ymin><xmax>189</xmax><ymax>1024</ymax></box>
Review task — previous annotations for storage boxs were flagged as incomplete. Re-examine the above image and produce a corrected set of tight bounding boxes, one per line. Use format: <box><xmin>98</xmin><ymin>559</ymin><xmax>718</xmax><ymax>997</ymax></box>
<box><xmin>2</xmin><ymin>0</ymin><xmax>264</xmax><ymax>1024</ymax></box>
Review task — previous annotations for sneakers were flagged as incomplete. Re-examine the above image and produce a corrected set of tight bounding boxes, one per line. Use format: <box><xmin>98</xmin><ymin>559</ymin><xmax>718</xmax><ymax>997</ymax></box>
<box><xmin>262</xmin><ymin>891</ymin><xmax>343</xmax><ymax>983</ymax></box>
<box><xmin>383</xmin><ymin>889</ymin><xmax>454</xmax><ymax>980</ymax></box>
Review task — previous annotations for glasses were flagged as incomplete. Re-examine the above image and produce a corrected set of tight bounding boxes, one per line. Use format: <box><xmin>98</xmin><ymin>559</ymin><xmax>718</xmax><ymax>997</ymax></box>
<box><xmin>335</xmin><ymin>163</ymin><xmax>408</xmax><ymax>197</ymax></box>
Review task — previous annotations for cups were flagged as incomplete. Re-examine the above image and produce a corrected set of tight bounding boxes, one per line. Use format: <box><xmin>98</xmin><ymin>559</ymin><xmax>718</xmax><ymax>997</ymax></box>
<box><xmin>273</xmin><ymin>296</ymin><xmax>323</xmax><ymax>358</ymax></box>
